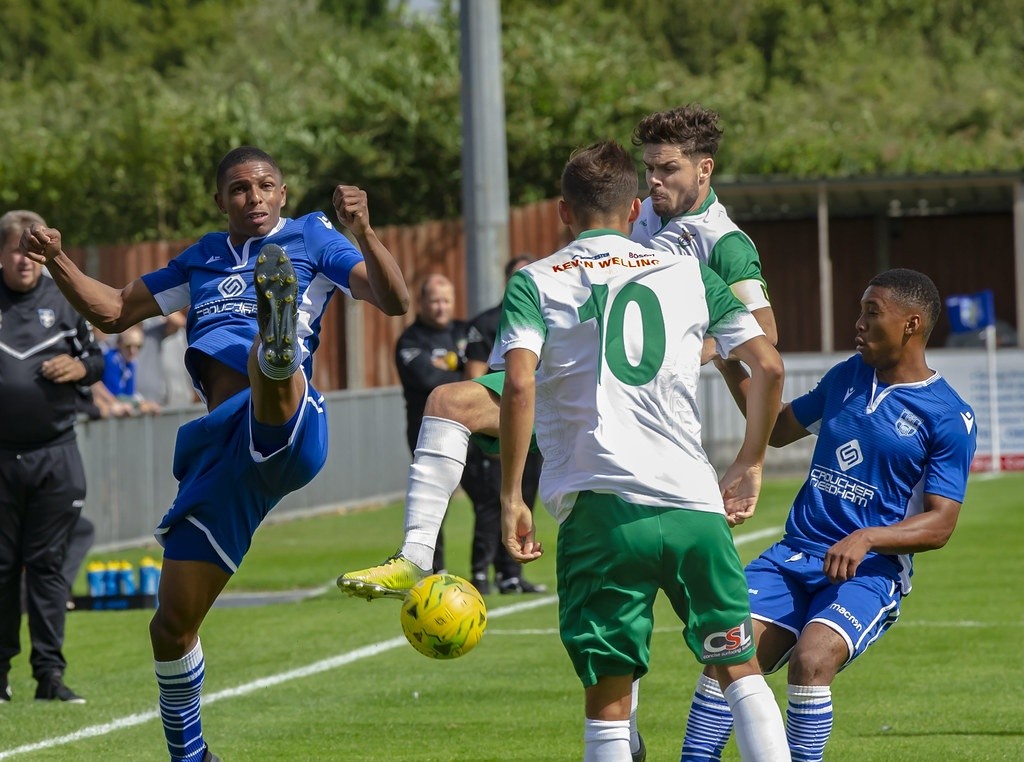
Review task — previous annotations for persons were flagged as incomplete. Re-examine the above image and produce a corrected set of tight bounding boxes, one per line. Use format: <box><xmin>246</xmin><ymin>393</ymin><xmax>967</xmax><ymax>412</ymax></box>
<box><xmin>489</xmin><ymin>141</ymin><xmax>792</xmax><ymax>762</ymax></box>
<box><xmin>338</xmin><ymin>102</ymin><xmax>778</xmax><ymax>602</ymax></box>
<box><xmin>19</xmin><ymin>146</ymin><xmax>411</xmax><ymax>735</ymax></box>
<box><xmin>680</xmin><ymin>269</ymin><xmax>978</xmax><ymax>762</ymax></box>
<box><xmin>395</xmin><ymin>256</ymin><xmax>547</xmax><ymax>593</ymax></box>
<box><xmin>0</xmin><ymin>208</ymin><xmax>104</xmax><ymax>705</ymax></box>
<box><xmin>73</xmin><ymin>321</ymin><xmax>161</xmax><ymax>419</ymax></box>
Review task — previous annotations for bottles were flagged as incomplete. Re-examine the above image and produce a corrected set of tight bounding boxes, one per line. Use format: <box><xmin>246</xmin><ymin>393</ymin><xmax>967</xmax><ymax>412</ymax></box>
<box><xmin>86</xmin><ymin>556</ymin><xmax>161</xmax><ymax>608</ymax></box>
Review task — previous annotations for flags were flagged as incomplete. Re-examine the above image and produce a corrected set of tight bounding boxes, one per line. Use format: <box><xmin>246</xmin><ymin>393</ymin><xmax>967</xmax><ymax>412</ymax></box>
<box><xmin>947</xmin><ymin>291</ymin><xmax>996</xmax><ymax>333</ymax></box>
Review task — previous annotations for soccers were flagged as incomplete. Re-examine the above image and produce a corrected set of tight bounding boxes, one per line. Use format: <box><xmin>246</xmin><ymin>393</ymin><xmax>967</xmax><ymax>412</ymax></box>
<box><xmin>399</xmin><ymin>572</ymin><xmax>489</xmax><ymax>661</ymax></box>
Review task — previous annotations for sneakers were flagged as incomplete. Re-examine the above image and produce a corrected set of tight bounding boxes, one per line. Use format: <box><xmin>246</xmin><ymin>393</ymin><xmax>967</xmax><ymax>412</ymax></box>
<box><xmin>336</xmin><ymin>548</ymin><xmax>434</xmax><ymax>603</ymax></box>
<box><xmin>253</xmin><ymin>243</ymin><xmax>296</xmax><ymax>368</ymax></box>
<box><xmin>34</xmin><ymin>682</ymin><xmax>86</xmax><ymax>704</ymax></box>
<box><xmin>499</xmin><ymin>575</ymin><xmax>546</xmax><ymax>596</ymax></box>
<box><xmin>470</xmin><ymin>574</ymin><xmax>490</xmax><ymax>595</ymax></box>
<box><xmin>202</xmin><ymin>741</ymin><xmax>219</xmax><ymax>762</ymax></box>
<box><xmin>632</xmin><ymin>731</ymin><xmax>647</xmax><ymax>762</ymax></box>
